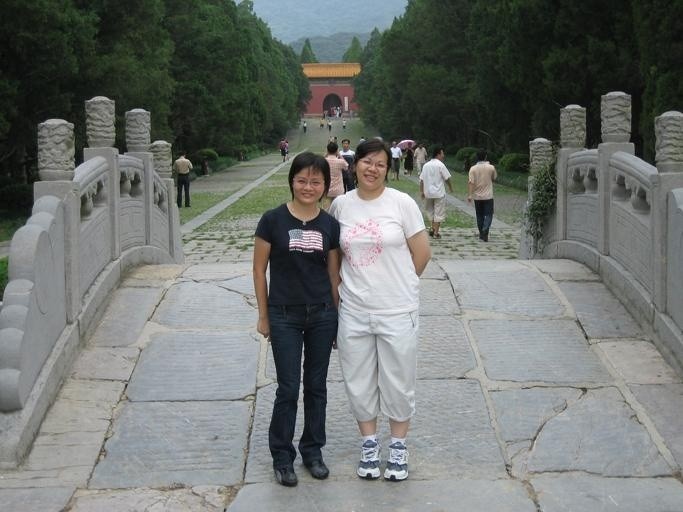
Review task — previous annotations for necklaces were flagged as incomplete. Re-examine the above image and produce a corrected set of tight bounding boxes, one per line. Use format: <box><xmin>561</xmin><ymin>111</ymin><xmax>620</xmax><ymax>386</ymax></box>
<box><xmin>287</xmin><ymin>202</ymin><xmax>318</xmax><ymax>226</ymax></box>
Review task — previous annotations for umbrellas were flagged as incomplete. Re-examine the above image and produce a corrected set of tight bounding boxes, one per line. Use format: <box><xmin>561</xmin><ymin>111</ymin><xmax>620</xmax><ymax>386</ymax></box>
<box><xmin>397</xmin><ymin>139</ymin><xmax>416</xmax><ymax>150</ymax></box>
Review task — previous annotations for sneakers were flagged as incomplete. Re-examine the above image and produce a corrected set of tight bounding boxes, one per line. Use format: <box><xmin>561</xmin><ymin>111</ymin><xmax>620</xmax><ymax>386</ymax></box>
<box><xmin>274</xmin><ymin>467</ymin><xmax>297</xmax><ymax>486</ymax></box>
<box><xmin>480</xmin><ymin>227</ymin><xmax>489</xmax><ymax>242</ymax></box>
<box><xmin>383</xmin><ymin>441</ymin><xmax>408</xmax><ymax>481</ymax></box>
<box><xmin>308</xmin><ymin>458</ymin><xmax>329</xmax><ymax>479</ymax></box>
<box><xmin>429</xmin><ymin>228</ymin><xmax>441</xmax><ymax>239</ymax></box>
<box><xmin>356</xmin><ymin>438</ymin><xmax>381</xmax><ymax>478</ymax></box>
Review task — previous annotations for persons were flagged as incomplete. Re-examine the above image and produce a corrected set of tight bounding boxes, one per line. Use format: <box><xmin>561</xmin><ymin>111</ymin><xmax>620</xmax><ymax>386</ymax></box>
<box><xmin>467</xmin><ymin>149</ymin><xmax>497</xmax><ymax>242</ymax></box>
<box><xmin>321</xmin><ymin>142</ymin><xmax>348</xmax><ymax>210</ymax></box>
<box><xmin>402</xmin><ymin>142</ymin><xmax>414</xmax><ymax>176</ymax></box>
<box><xmin>326</xmin><ymin>138</ymin><xmax>431</xmax><ymax>482</ymax></box>
<box><xmin>251</xmin><ymin>152</ymin><xmax>341</xmax><ymax>486</ymax></box>
<box><xmin>338</xmin><ymin>139</ymin><xmax>356</xmax><ymax>193</ymax></box>
<box><xmin>278</xmin><ymin>104</ymin><xmax>366</xmax><ymax>162</ymax></box>
<box><xmin>389</xmin><ymin>141</ymin><xmax>403</xmax><ymax>182</ymax></box>
<box><xmin>419</xmin><ymin>146</ymin><xmax>454</xmax><ymax>239</ymax></box>
<box><xmin>413</xmin><ymin>142</ymin><xmax>428</xmax><ymax>177</ymax></box>
<box><xmin>172</xmin><ymin>151</ymin><xmax>193</xmax><ymax>207</ymax></box>
